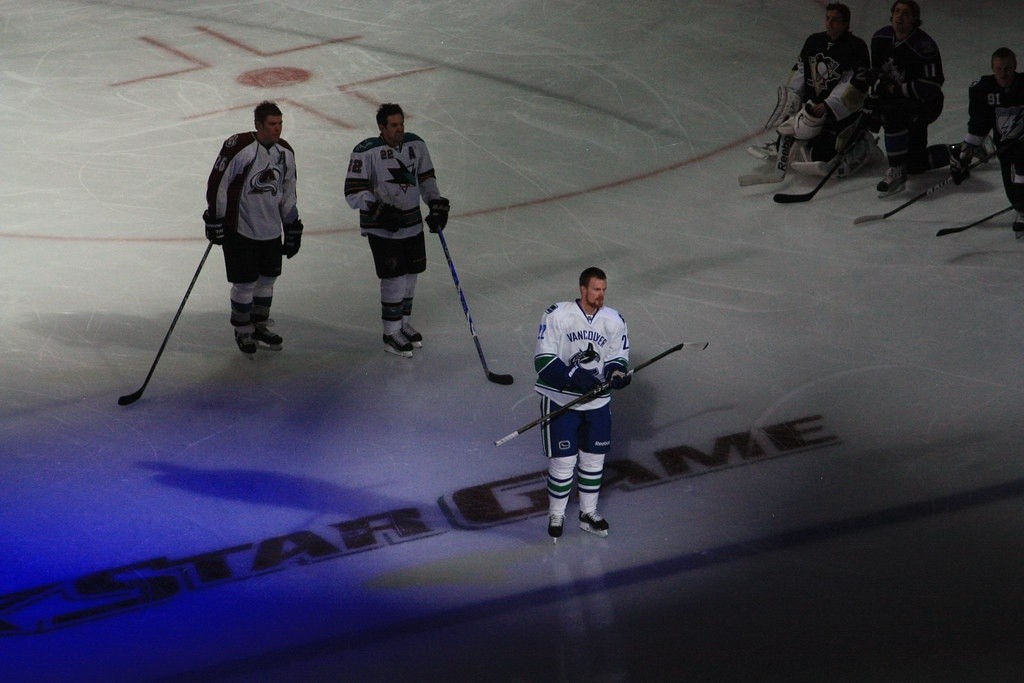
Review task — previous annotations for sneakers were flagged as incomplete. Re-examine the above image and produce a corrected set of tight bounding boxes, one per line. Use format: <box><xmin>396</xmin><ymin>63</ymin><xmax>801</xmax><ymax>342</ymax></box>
<box><xmin>383</xmin><ymin>332</ymin><xmax>414</xmax><ymax>357</ymax></box>
<box><xmin>579</xmin><ymin>510</ymin><xmax>608</xmax><ymax>537</ymax></box>
<box><xmin>401</xmin><ymin>325</ymin><xmax>423</xmax><ymax>348</ymax></box>
<box><xmin>1013</xmin><ymin>211</ymin><xmax>1024</xmax><ymax>239</ymax></box>
<box><xmin>252</xmin><ymin>324</ymin><xmax>283</xmax><ymax>350</ymax></box>
<box><xmin>876</xmin><ymin>165</ymin><xmax>907</xmax><ymax>197</ymax></box>
<box><xmin>234</xmin><ymin>329</ymin><xmax>256</xmax><ymax>360</ymax></box>
<box><xmin>974</xmin><ymin>135</ymin><xmax>995</xmax><ymax>162</ymax></box>
<box><xmin>547</xmin><ymin>515</ymin><xmax>565</xmax><ymax>544</ymax></box>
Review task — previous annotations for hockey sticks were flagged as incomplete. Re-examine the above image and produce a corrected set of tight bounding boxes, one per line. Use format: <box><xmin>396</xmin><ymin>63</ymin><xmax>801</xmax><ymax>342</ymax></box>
<box><xmin>437</xmin><ymin>225</ymin><xmax>514</xmax><ymax>386</ymax></box>
<box><xmin>853</xmin><ymin>134</ymin><xmax>1024</xmax><ymax>225</ymax></box>
<box><xmin>738</xmin><ymin>135</ymin><xmax>794</xmax><ymax>186</ymax></box>
<box><xmin>936</xmin><ymin>202</ymin><xmax>1023</xmax><ymax>236</ymax></box>
<box><xmin>773</xmin><ymin>130</ymin><xmax>871</xmax><ymax>203</ymax></box>
<box><xmin>493</xmin><ymin>340</ymin><xmax>713</xmax><ymax>447</ymax></box>
<box><xmin>119</xmin><ymin>238</ymin><xmax>215</xmax><ymax>407</ymax></box>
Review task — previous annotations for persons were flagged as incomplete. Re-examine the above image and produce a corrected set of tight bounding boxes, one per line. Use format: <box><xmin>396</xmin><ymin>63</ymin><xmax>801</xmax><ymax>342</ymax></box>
<box><xmin>203</xmin><ymin>100</ymin><xmax>303</xmax><ymax>358</ymax></box>
<box><xmin>344</xmin><ymin>102</ymin><xmax>450</xmax><ymax>357</ymax></box>
<box><xmin>862</xmin><ymin>0</ymin><xmax>996</xmax><ymax>198</ymax></box>
<box><xmin>748</xmin><ymin>2</ymin><xmax>884</xmax><ymax>178</ymax></box>
<box><xmin>950</xmin><ymin>47</ymin><xmax>1024</xmax><ymax>238</ymax></box>
<box><xmin>534</xmin><ymin>266</ymin><xmax>630</xmax><ymax>544</ymax></box>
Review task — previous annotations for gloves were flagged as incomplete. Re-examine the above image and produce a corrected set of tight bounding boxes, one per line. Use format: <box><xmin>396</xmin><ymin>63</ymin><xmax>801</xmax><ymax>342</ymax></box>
<box><xmin>282</xmin><ymin>219</ymin><xmax>303</xmax><ymax>259</ymax></box>
<box><xmin>565</xmin><ymin>363</ymin><xmax>601</xmax><ymax>395</ymax></box>
<box><xmin>203</xmin><ymin>209</ymin><xmax>227</xmax><ymax>245</ymax></box>
<box><xmin>603</xmin><ymin>357</ymin><xmax>632</xmax><ymax>389</ymax></box>
<box><xmin>368</xmin><ymin>200</ymin><xmax>404</xmax><ymax>233</ymax></box>
<box><xmin>950</xmin><ymin>152</ymin><xmax>972</xmax><ymax>185</ymax></box>
<box><xmin>425</xmin><ymin>197</ymin><xmax>451</xmax><ymax>233</ymax></box>
